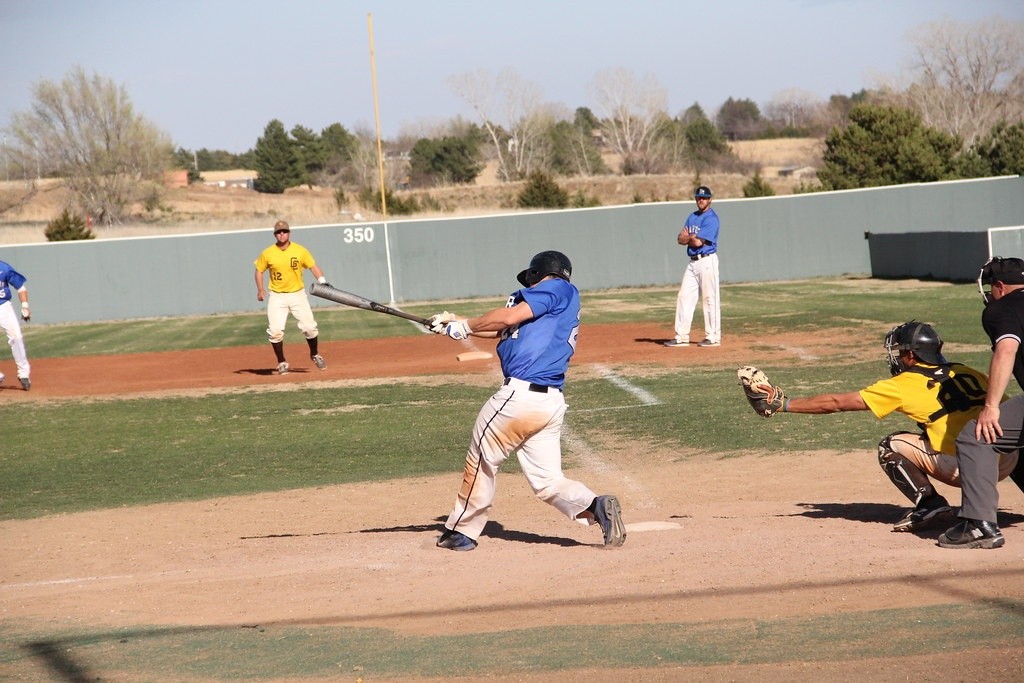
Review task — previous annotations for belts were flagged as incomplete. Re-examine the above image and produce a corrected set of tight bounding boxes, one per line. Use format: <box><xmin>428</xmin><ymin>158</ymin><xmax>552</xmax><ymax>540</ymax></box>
<box><xmin>503</xmin><ymin>378</ymin><xmax>563</xmax><ymax>393</ymax></box>
<box><xmin>691</xmin><ymin>254</ymin><xmax>709</xmax><ymax>260</ymax></box>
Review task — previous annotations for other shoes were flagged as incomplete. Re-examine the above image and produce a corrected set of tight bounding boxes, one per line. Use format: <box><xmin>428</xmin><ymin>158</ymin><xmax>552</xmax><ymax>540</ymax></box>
<box><xmin>276</xmin><ymin>362</ymin><xmax>289</xmax><ymax>376</ymax></box>
<box><xmin>664</xmin><ymin>339</ymin><xmax>689</xmax><ymax>347</ymax></box>
<box><xmin>19</xmin><ymin>378</ymin><xmax>30</xmax><ymax>391</ymax></box>
<box><xmin>311</xmin><ymin>354</ymin><xmax>327</xmax><ymax>371</ymax></box>
<box><xmin>698</xmin><ymin>338</ymin><xmax>721</xmax><ymax>347</ymax></box>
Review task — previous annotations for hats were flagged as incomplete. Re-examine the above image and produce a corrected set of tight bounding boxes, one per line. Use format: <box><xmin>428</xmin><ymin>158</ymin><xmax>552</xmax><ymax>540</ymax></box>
<box><xmin>274</xmin><ymin>220</ymin><xmax>290</xmax><ymax>234</ymax></box>
<box><xmin>991</xmin><ymin>258</ymin><xmax>1024</xmax><ymax>284</ymax></box>
<box><xmin>695</xmin><ymin>187</ymin><xmax>712</xmax><ymax>198</ymax></box>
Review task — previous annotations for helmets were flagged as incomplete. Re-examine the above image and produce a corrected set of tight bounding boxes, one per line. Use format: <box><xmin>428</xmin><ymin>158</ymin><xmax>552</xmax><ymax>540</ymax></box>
<box><xmin>517</xmin><ymin>251</ymin><xmax>572</xmax><ymax>288</ymax></box>
<box><xmin>896</xmin><ymin>322</ymin><xmax>946</xmax><ymax>366</ymax></box>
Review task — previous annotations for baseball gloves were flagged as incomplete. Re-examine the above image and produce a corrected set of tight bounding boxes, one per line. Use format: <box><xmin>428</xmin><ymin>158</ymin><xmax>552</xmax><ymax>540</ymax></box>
<box><xmin>735</xmin><ymin>361</ymin><xmax>786</xmax><ymax>421</ymax></box>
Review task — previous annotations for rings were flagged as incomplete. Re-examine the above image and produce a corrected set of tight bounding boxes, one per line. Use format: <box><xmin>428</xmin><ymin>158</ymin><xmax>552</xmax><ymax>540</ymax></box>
<box><xmin>988</xmin><ymin>428</ymin><xmax>995</xmax><ymax>430</ymax></box>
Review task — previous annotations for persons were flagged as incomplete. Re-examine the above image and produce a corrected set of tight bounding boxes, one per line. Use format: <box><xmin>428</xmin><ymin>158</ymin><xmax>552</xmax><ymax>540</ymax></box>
<box><xmin>0</xmin><ymin>260</ymin><xmax>32</xmax><ymax>391</ymax></box>
<box><xmin>424</xmin><ymin>250</ymin><xmax>627</xmax><ymax>552</ymax></box>
<box><xmin>254</xmin><ymin>221</ymin><xmax>334</xmax><ymax>376</ymax></box>
<box><xmin>664</xmin><ymin>186</ymin><xmax>721</xmax><ymax>347</ymax></box>
<box><xmin>937</xmin><ymin>256</ymin><xmax>1024</xmax><ymax>550</ymax></box>
<box><xmin>737</xmin><ymin>318</ymin><xmax>1019</xmax><ymax>532</ymax></box>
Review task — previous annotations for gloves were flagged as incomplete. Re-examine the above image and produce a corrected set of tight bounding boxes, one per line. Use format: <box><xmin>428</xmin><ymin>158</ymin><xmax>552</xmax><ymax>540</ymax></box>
<box><xmin>20</xmin><ymin>302</ymin><xmax>32</xmax><ymax>321</ymax></box>
<box><xmin>426</xmin><ymin>311</ymin><xmax>473</xmax><ymax>341</ymax></box>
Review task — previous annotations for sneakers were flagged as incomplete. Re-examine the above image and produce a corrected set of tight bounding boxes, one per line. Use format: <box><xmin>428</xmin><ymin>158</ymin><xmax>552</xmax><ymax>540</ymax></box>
<box><xmin>593</xmin><ymin>495</ymin><xmax>627</xmax><ymax>550</ymax></box>
<box><xmin>439</xmin><ymin>533</ymin><xmax>479</xmax><ymax>552</ymax></box>
<box><xmin>893</xmin><ymin>494</ymin><xmax>953</xmax><ymax>533</ymax></box>
<box><xmin>939</xmin><ymin>519</ymin><xmax>1005</xmax><ymax>550</ymax></box>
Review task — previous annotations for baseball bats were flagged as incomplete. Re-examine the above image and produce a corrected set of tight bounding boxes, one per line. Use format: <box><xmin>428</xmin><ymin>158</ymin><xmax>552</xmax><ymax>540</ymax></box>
<box><xmin>308</xmin><ymin>282</ymin><xmax>446</xmax><ymax>327</ymax></box>
